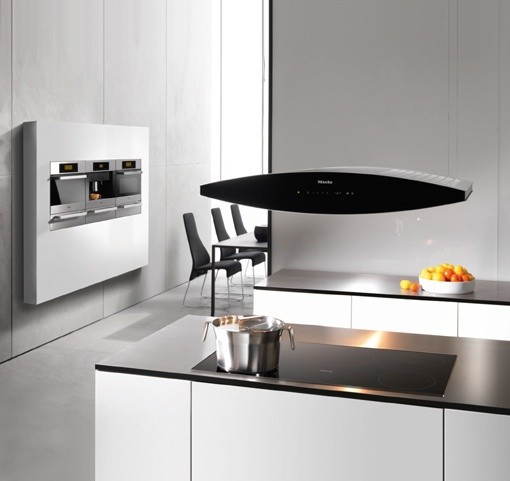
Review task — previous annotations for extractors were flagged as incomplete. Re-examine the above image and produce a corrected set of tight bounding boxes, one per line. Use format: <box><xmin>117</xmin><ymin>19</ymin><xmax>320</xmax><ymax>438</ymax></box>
<box><xmin>199</xmin><ymin>168</ymin><xmax>473</xmax><ymax>217</ymax></box>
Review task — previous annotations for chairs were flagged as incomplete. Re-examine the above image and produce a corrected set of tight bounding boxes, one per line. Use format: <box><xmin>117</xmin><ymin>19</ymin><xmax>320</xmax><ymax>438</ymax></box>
<box><xmin>230</xmin><ymin>204</ymin><xmax>268</xmax><ymax>287</ymax></box>
<box><xmin>210</xmin><ymin>208</ymin><xmax>267</xmax><ymax>287</ymax></box>
<box><xmin>182</xmin><ymin>212</ymin><xmax>245</xmax><ymax>309</ymax></box>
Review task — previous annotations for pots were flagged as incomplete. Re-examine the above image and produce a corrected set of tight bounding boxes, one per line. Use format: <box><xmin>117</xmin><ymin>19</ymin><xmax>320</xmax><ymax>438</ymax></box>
<box><xmin>202</xmin><ymin>315</ymin><xmax>295</xmax><ymax>374</ymax></box>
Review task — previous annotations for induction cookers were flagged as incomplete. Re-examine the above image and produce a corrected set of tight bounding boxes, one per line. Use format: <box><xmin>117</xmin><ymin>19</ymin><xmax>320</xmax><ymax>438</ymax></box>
<box><xmin>192</xmin><ymin>339</ymin><xmax>458</xmax><ymax>399</ymax></box>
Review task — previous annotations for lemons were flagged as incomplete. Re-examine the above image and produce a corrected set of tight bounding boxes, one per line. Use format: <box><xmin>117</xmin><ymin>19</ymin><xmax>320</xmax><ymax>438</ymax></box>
<box><xmin>400</xmin><ymin>279</ymin><xmax>410</xmax><ymax>290</ymax></box>
<box><xmin>420</xmin><ymin>262</ymin><xmax>442</xmax><ymax>280</ymax></box>
<box><xmin>443</xmin><ymin>264</ymin><xmax>468</xmax><ymax>275</ymax></box>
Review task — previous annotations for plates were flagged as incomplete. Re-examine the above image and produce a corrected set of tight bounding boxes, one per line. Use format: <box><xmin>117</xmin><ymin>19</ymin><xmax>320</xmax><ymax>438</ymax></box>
<box><xmin>418</xmin><ymin>277</ymin><xmax>474</xmax><ymax>295</ymax></box>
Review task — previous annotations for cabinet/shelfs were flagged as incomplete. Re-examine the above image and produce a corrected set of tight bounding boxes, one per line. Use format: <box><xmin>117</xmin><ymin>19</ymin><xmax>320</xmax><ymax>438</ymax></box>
<box><xmin>351</xmin><ymin>296</ymin><xmax>457</xmax><ymax>341</ymax></box>
<box><xmin>458</xmin><ymin>303</ymin><xmax>510</xmax><ymax>342</ymax></box>
<box><xmin>252</xmin><ymin>290</ymin><xmax>351</xmax><ymax>330</ymax></box>
<box><xmin>444</xmin><ymin>409</ymin><xmax>510</xmax><ymax>481</ymax></box>
<box><xmin>94</xmin><ymin>370</ymin><xmax>191</xmax><ymax>481</ymax></box>
<box><xmin>191</xmin><ymin>381</ymin><xmax>443</xmax><ymax>481</ymax></box>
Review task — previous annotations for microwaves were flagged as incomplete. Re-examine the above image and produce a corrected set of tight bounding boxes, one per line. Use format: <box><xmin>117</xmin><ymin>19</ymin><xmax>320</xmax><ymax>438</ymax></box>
<box><xmin>51</xmin><ymin>161</ymin><xmax>142</xmax><ymax>230</ymax></box>
